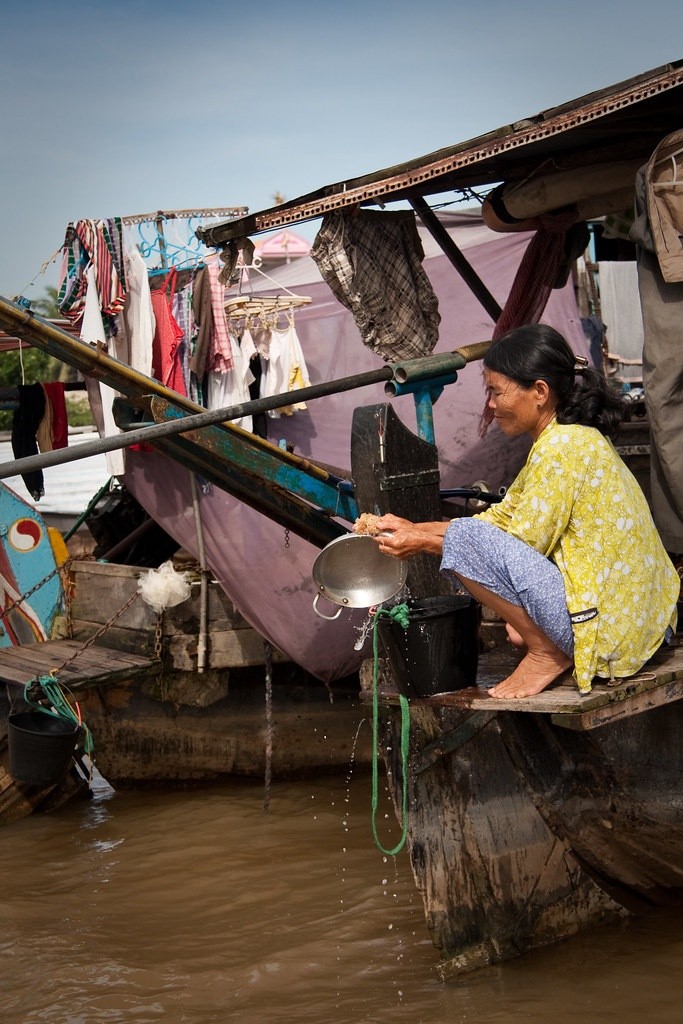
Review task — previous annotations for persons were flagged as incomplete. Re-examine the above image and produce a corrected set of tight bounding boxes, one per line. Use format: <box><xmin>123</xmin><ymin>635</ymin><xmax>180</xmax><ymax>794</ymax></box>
<box><xmin>372</xmin><ymin>325</ymin><xmax>681</xmax><ymax>699</ymax></box>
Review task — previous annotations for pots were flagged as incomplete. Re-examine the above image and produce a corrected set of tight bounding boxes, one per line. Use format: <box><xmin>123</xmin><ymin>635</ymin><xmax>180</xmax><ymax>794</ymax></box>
<box><xmin>312</xmin><ymin>531</ymin><xmax>410</xmax><ymax>620</ymax></box>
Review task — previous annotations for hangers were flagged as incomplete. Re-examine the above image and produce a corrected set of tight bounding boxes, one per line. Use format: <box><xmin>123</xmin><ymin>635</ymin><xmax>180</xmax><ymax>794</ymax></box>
<box><xmin>65</xmin><ymin>242</ymin><xmax>93</xmax><ymax>282</ymax></box>
<box><xmin>136</xmin><ymin>213</ymin><xmax>222</xmax><ymax>279</ymax></box>
<box><xmin>651</xmin><ymin>147</ymin><xmax>683</xmax><ymax>186</ymax></box>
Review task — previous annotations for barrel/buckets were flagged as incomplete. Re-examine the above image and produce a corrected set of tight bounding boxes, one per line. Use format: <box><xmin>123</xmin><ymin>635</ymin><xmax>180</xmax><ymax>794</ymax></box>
<box><xmin>6</xmin><ymin>678</ymin><xmax>81</xmax><ymax>785</ymax></box>
<box><xmin>369</xmin><ymin>595</ymin><xmax>482</xmax><ymax>698</ymax></box>
